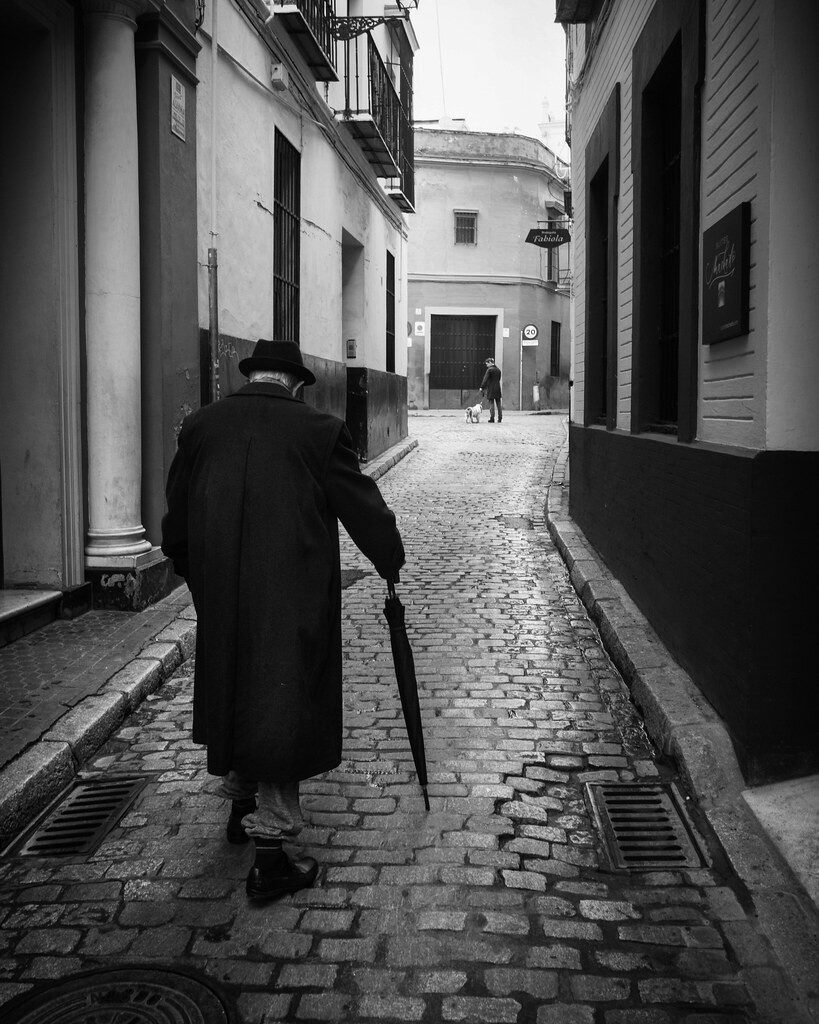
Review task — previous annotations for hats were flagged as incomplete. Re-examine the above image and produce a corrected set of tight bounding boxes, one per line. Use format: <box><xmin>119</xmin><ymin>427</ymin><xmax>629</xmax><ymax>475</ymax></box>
<box><xmin>238</xmin><ymin>339</ymin><xmax>316</xmax><ymax>387</ymax></box>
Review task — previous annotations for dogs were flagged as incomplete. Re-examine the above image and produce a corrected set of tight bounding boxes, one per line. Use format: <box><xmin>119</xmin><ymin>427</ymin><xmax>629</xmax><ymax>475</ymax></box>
<box><xmin>465</xmin><ymin>401</ymin><xmax>482</xmax><ymax>423</ymax></box>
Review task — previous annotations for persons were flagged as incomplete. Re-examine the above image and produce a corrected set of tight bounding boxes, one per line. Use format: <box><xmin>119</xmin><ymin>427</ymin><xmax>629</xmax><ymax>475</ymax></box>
<box><xmin>479</xmin><ymin>358</ymin><xmax>503</xmax><ymax>423</ymax></box>
<box><xmin>162</xmin><ymin>340</ymin><xmax>408</xmax><ymax>906</ymax></box>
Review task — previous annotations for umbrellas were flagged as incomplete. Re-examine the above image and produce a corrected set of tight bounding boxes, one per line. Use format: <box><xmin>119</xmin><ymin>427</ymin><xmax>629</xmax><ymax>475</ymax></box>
<box><xmin>380</xmin><ymin>578</ymin><xmax>434</xmax><ymax>814</ymax></box>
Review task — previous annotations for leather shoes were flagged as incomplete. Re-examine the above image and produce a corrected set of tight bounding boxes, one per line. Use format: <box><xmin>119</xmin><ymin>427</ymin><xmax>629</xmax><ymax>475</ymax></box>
<box><xmin>226</xmin><ymin>799</ymin><xmax>257</xmax><ymax>844</ymax></box>
<box><xmin>246</xmin><ymin>856</ymin><xmax>319</xmax><ymax>899</ymax></box>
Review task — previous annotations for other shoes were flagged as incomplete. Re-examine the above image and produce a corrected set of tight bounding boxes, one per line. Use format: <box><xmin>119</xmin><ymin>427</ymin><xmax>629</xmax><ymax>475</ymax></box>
<box><xmin>488</xmin><ymin>420</ymin><xmax>494</xmax><ymax>423</ymax></box>
<box><xmin>498</xmin><ymin>419</ymin><xmax>502</xmax><ymax>422</ymax></box>
